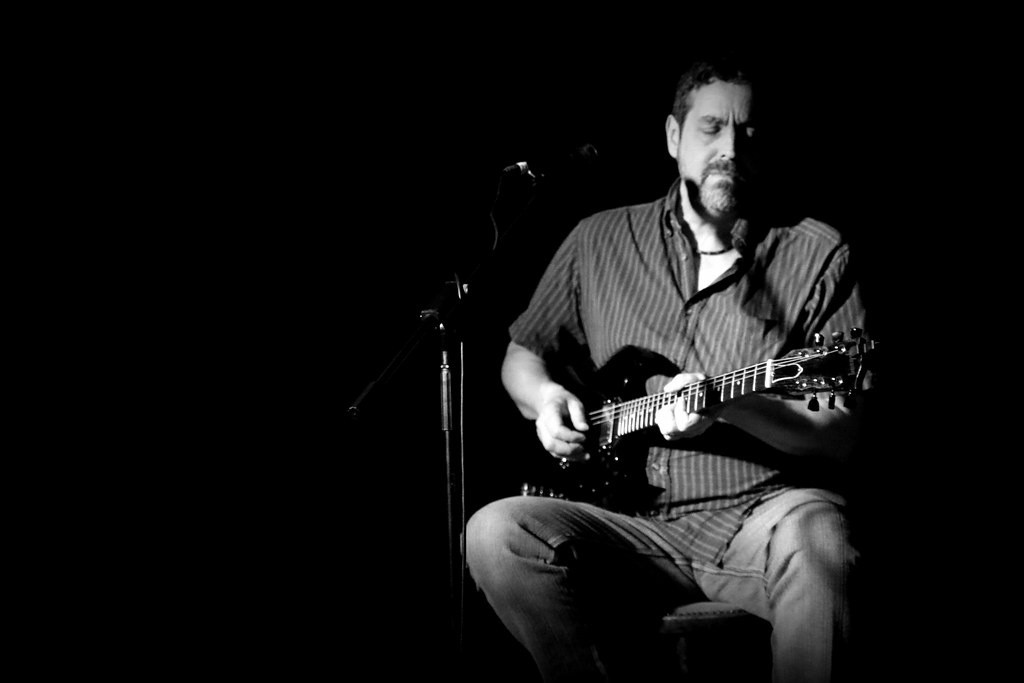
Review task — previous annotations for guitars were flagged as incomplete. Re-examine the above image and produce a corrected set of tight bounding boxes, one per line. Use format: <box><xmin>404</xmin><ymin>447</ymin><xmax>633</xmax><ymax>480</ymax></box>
<box><xmin>531</xmin><ymin>327</ymin><xmax>876</xmax><ymax>508</ymax></box>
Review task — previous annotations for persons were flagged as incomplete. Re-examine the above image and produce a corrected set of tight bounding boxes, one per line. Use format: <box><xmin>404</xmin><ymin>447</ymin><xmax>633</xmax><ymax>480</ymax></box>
<box><xmin>458</xmin><ymin>54</ymin><xmax>874</xmax><ymax>683</ymax></box>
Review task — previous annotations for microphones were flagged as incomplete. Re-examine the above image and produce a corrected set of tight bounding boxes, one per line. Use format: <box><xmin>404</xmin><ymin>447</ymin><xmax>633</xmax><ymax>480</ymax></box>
<box><xmin>531</xmin><ymin>143</ymin><xmax>598</xmax><ymax>186</ymax></box>
